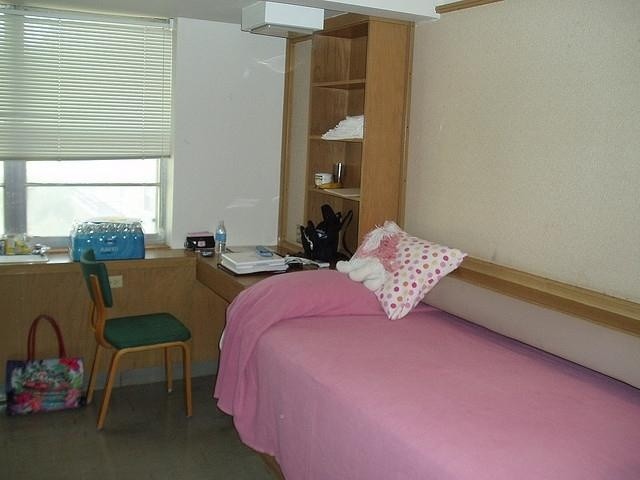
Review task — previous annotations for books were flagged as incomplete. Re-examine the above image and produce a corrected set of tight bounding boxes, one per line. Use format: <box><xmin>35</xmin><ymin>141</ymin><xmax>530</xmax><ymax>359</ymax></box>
<box><xmin>221</xmin><ymin>252</ymin><xmax>286</xmax><ymax>274</ymax></box>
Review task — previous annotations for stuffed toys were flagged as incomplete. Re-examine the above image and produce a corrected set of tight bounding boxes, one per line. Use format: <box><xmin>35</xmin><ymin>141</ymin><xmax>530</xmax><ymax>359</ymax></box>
<box><xmin>336</xmin><ymin>220</ymin><xmax>403</xmax><ymax>291</ymax></box>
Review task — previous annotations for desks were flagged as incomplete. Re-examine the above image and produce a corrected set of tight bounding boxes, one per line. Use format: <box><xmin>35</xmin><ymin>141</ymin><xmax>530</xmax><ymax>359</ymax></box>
<box><xmin>198</xmin><ymin>245</ymin><xmax>331</xmax><ymax>305</ymax></box>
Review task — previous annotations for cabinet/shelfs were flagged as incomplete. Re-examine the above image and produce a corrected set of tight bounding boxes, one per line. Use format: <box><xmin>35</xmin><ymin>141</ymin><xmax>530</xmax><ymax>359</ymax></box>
<box><xmin>282</xmin><ymin>15</ymin><xmax>412</xmax><ymax>262</ymax></box>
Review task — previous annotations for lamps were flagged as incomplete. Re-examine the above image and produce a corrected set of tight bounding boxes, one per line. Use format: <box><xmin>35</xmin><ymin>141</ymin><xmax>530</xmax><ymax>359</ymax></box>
<box><xmin>239</xmin><ymin>1</ymin><xmax>325</xmax><ymax>39</ymax></box>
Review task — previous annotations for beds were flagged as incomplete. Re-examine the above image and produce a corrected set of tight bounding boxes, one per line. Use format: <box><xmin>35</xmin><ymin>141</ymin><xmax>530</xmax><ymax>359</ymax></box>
<box><xmin>226</xmin><ymin>267</ymin><xmax>638</xmax><ymax>476</ymax></box>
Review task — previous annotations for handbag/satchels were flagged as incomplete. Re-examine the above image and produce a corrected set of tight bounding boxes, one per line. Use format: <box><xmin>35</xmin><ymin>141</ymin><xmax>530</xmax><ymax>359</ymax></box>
<box><xmin>3</xmin><ymin>315</ymin><xmax>85</xmax><ymax>416</ymax></box>
<box><xmin>299</xmin><ymin>205</ymin><xmax>355</xmax><ymax>262</ymax></box>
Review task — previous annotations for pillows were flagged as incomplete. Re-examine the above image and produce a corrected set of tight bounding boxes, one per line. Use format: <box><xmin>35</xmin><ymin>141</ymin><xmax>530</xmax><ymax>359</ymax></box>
<box><xmin>255</xmin><ymin>222</ymin><xmax>471</xmax><ymax>331</ymax></box>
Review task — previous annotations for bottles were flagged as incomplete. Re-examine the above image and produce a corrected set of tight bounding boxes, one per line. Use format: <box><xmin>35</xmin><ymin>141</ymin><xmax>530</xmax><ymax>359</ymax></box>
<box><xmin>71</xmin><ymin>220</ymin><xmax>144</xmax><ymax>264</ymax></box>
<box><xmin>1</xmin><ymin>233</ymin><xmax>18</xmax><ymax>256</ymax></box>
<box><xmin>215</xmin><ymin>220</ymin><xmax>227</xmax><ymax>254</ymax></box>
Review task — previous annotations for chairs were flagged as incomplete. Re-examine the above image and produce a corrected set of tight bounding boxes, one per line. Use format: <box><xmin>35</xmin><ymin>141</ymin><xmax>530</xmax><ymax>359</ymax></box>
<box><xmin>81</xmin><ymin>250</ymin><xmax>192</xmax><ymax>429</ymax></box>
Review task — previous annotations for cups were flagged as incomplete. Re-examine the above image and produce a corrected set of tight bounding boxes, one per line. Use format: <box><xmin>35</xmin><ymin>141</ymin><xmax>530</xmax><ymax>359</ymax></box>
<box><xmin>334</xmin><ymin>162</ymin><xmax>344</xmax><ymax>183</ymax></box>
